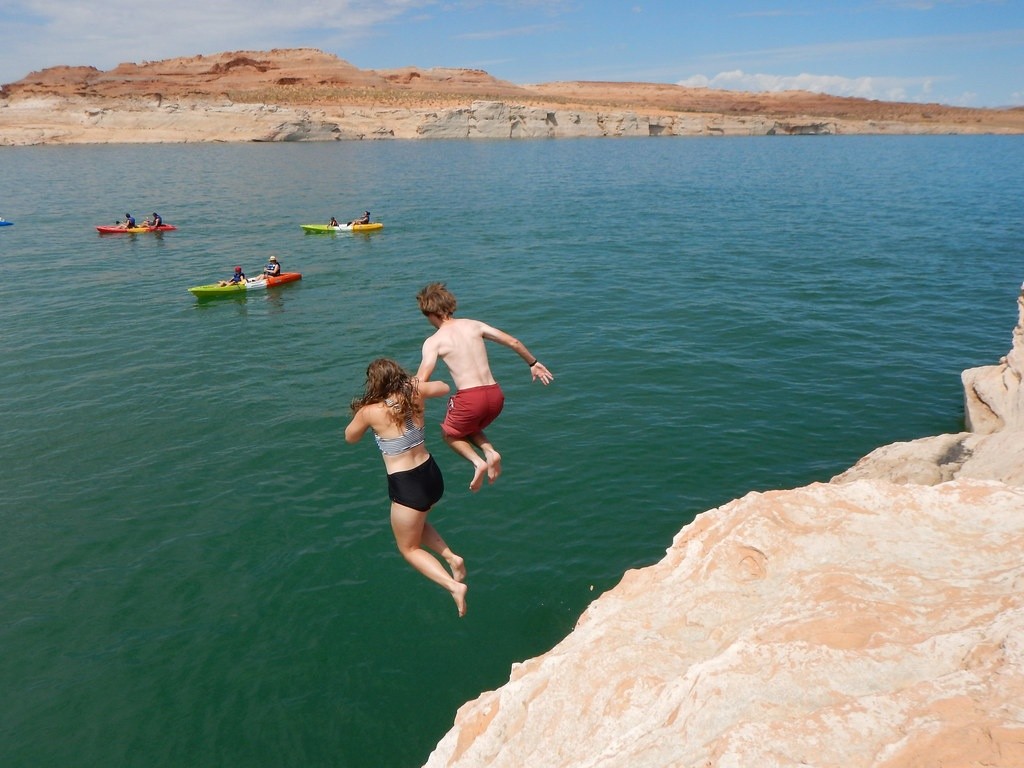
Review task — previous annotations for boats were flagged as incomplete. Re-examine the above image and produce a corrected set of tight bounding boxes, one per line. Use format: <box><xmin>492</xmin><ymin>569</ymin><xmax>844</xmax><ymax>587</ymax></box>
<box><xmin>187</xmin><ymin>272</ymin><xmax>302</xmax><ymax>298</ymax></box>
<box><xmin>299</xmin><ymin>223</ymin><xmax>384</xmax><ymax>235</ymax></box>
<box><xmin>95</xmin><ymin>224</ymin><xmax>177</xmax><ymax>233</ymax></box>
<box><xmin>0</xmin><ymin>217</ymin><xmax>14</xmax><ymax>226</ymax></box>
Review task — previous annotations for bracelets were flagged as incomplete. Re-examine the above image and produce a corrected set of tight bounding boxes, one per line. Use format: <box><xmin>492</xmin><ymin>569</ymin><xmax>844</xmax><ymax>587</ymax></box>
<box><xmin>529</xmin><ymin>360</ymin><xmax>537</xmax><ymax>367</ymax></box>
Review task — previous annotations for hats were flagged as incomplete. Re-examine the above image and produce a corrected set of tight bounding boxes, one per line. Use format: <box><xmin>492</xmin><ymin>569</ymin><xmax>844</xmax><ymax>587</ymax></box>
<box><xmin>269</xmin><ymin>256</ymin><xmax>276</xmax><ymax>261</ymax></box>
<box><xmin>235</xmin><ymin>266</ymin><xmax>241</xmax><ymax>272</ymax></box>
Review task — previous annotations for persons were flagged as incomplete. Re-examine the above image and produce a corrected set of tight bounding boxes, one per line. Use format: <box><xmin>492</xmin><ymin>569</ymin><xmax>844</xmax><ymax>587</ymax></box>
<box><xmin>218</xmin><ymin>256</ymin><xmax>280</xmax><ymax>287</ymax></box>
<box><xmin>412</xmin><ymin>283</ymin><xmax>554</xmax><ymax>492</ymax></box>
<box><xmin>330</xmin><ymin>211</ymin><xmax>370</xmax><ymax>226</ymax></box>
<box><xmin>344</xmin><ymin>359</ymin><xmax>468</xmax><ymax>618</ymax></box>
<box><xmin>120</xmin><ymin>213</ymin><xmax>162</xmax><ymax>227</ymax></box>
